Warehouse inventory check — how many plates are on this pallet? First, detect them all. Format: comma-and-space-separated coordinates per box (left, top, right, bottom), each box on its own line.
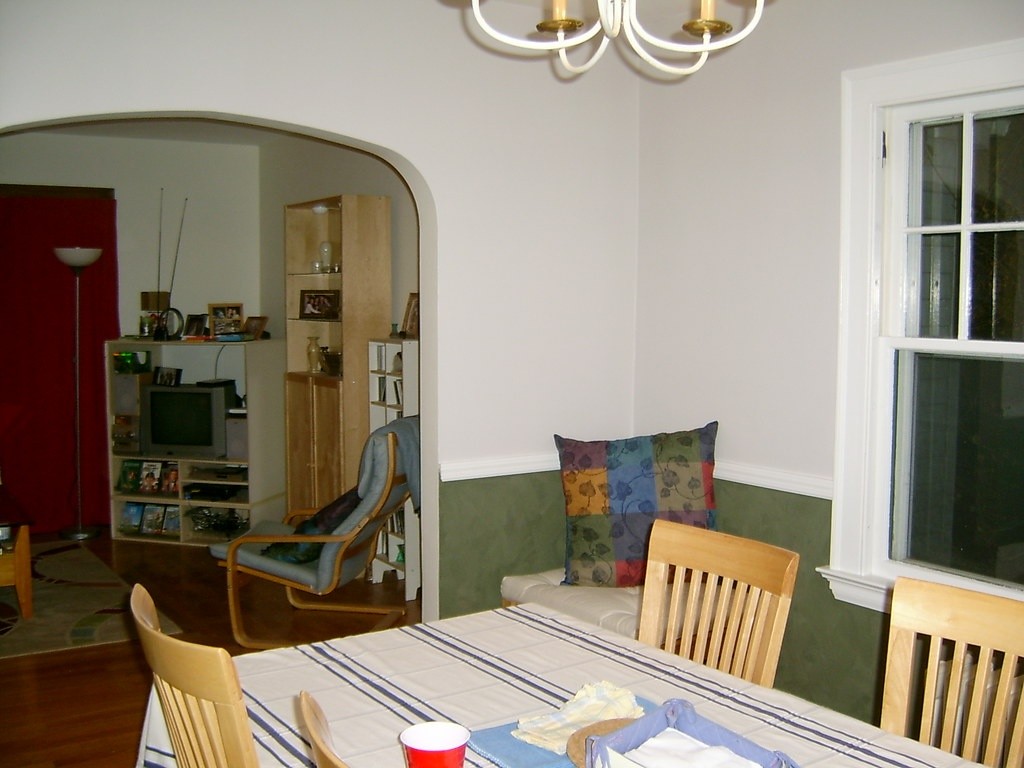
566, 718, 641, 767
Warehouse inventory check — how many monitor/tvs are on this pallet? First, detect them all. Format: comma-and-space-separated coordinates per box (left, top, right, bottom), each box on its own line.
141, 384, 227, 460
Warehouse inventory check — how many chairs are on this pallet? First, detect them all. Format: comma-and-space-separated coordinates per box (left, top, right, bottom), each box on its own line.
129, 584, 260, 768
210, 415, 420, 648
637, 520, 800, 688
880, 576, 1024, 768
299, 689, 350, 768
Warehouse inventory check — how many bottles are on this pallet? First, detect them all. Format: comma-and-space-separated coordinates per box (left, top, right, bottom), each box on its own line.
307, 337, 321, 372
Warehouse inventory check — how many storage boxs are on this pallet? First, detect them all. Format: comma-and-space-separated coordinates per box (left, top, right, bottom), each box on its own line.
113, 352, 152, 374
586, 698, 802, 768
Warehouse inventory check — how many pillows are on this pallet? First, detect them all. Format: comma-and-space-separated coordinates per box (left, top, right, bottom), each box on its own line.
554, 420, 718, 588
261, 484, 363, 565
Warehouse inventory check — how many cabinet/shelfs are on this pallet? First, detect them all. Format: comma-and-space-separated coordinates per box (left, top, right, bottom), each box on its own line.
283, 195, 393, 528
366, 338, 422, 600
104, 338, 283, 547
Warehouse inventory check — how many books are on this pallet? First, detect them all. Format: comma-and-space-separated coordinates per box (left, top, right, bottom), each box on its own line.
117, 461, 180, 537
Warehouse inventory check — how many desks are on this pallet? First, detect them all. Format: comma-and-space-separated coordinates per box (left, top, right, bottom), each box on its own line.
133, 601, 994, 767
0, 485, 35, 620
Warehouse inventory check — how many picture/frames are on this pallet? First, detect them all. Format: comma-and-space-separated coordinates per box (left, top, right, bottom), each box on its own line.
182, 314, 207, 337
243, 316, 269, 339
402, 293, 419, 340
208, 303, 244, 336
299, 289, 342, 320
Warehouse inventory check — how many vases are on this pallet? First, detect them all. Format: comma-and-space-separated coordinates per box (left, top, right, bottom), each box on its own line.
307, 337, 321, 373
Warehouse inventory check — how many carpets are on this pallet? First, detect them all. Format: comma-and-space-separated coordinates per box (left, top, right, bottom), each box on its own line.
0, 540, 182, 661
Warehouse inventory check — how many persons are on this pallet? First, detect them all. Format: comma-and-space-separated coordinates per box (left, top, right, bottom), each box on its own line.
159, 371, 174, 385
215, 309, 240, 332
304, 296, 333, 314
162, 468, 179, 493
140, 472, 157, 491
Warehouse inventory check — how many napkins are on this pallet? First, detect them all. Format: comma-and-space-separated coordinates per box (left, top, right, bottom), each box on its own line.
624, 728, 761, 767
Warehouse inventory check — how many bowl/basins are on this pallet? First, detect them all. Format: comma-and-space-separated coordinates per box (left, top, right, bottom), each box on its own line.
318, 352, 342, 375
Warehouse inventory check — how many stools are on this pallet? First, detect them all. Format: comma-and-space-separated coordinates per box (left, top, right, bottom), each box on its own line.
500, 567, 762, 660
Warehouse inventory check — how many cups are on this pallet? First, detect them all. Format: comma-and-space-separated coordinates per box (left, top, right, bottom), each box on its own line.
400, 722, 470, 768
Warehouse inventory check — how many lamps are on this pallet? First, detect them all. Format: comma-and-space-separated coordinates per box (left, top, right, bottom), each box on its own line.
312, 206, 328, 215
54, 247, 104, 543
140, 292, 170, 335
472, 0, 765, 76
